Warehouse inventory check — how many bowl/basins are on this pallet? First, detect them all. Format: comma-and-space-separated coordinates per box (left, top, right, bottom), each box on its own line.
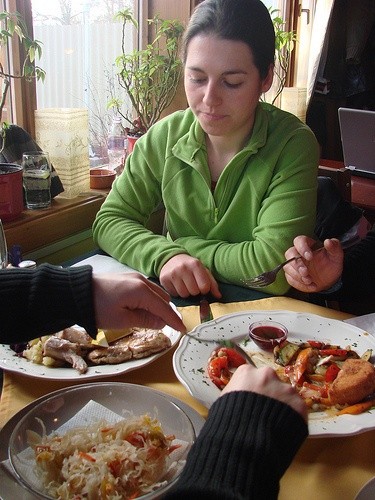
249, 321, 289, 350
90, 169, 116, 188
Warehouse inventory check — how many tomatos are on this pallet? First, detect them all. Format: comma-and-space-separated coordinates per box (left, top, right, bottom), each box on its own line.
208, 346, 246, 390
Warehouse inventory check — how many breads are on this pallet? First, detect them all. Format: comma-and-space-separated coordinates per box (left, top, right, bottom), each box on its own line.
18, 324, 171, 373
327, 358, 375, 404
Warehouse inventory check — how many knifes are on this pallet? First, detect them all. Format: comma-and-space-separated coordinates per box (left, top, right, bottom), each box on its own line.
199, 294, 214, 324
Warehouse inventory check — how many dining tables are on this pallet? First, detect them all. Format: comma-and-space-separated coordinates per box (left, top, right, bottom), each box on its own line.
0, 249, 375, 500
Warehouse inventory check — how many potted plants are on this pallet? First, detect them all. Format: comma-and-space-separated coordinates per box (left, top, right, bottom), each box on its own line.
106, 8, 186, 153
0, 10, 47, 221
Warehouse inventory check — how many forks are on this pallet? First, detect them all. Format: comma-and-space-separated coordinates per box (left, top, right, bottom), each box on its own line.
239, 226, 361, 288
181, 326, 258, 368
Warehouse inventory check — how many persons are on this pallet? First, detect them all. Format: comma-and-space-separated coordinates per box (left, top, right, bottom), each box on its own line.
283, 231, 375, 301
91, 0, 319, 297
0, 265, 309, 500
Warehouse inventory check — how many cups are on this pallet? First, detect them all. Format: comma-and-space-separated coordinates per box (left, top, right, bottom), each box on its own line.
22, 151, 51, 210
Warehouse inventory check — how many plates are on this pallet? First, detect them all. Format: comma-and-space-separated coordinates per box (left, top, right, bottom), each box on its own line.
1, 300, 185, 380
173, 309, 374, 440
0, 382, 209, 500
8, 381, 198, 500
